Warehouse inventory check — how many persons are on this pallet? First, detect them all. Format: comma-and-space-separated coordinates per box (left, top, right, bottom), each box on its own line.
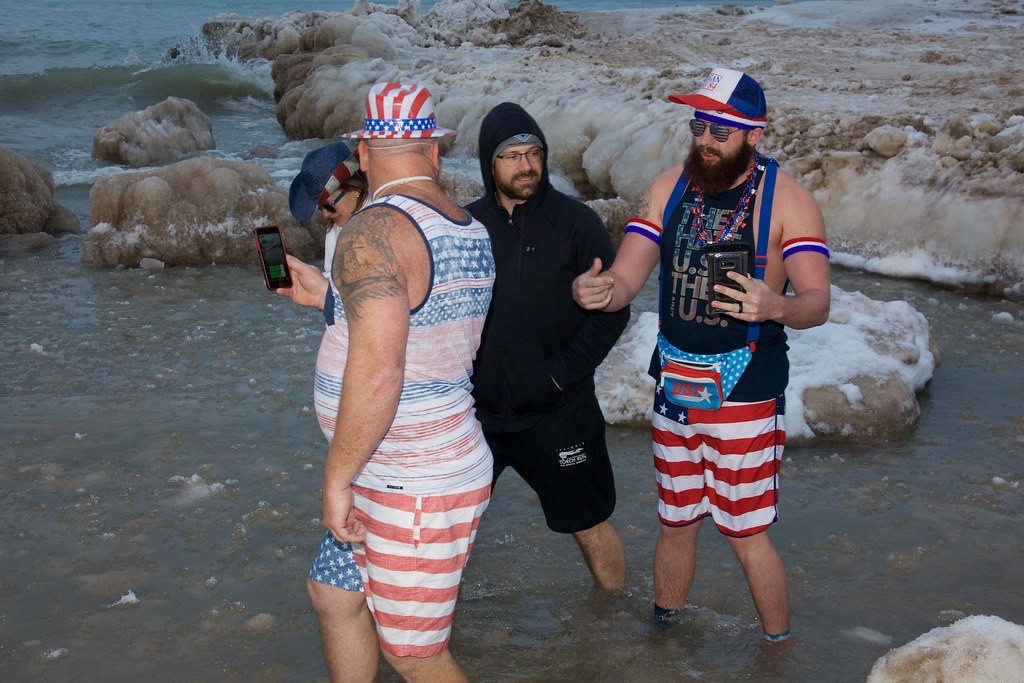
262, 77, 498, 683
456, 99, 634, 593
571, 67, 834, 645
289, 140, 378, 275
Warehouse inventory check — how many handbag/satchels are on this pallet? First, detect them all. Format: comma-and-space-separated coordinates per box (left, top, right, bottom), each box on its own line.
655, 332, 788, 412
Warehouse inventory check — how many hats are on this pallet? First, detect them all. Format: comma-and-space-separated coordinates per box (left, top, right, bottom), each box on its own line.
668, 68, 767, 119
340, 82, 458, 140
288, 144, 360, 222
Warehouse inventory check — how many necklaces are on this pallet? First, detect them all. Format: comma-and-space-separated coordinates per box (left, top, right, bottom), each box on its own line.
371, 173, 435, 203
688, 151, 761, 249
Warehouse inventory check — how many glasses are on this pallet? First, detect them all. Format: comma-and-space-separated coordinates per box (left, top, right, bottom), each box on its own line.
689, 119, 742, 143
497, 148, 544, 167
317, 190, 347, 214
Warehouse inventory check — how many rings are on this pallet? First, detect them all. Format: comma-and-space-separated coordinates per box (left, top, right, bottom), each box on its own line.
738, 302, 743, 314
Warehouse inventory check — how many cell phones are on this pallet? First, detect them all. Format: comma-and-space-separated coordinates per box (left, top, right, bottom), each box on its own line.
254, 226, 293, 290
708, 242, 753, 315
